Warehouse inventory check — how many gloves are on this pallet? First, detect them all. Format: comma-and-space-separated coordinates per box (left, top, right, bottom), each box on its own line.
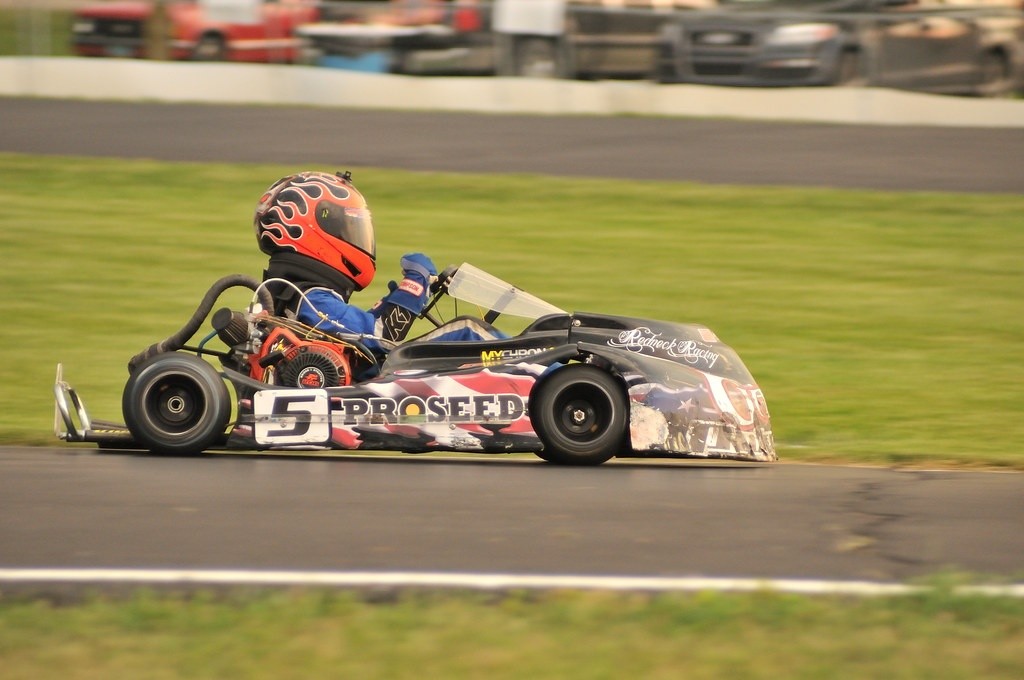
387, 253, 436, 316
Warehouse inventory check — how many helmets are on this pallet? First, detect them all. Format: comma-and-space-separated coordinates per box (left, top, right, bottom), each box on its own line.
253, 171, 376, 292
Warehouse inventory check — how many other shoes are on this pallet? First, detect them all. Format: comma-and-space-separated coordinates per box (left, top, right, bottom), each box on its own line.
585, 352, 612, 372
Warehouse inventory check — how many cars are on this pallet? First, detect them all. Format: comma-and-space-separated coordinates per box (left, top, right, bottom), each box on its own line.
70, 0, 446, 63
653, 0, 1022, 99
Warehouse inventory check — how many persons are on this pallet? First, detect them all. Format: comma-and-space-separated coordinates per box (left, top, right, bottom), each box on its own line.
254, 171, 513, 383
